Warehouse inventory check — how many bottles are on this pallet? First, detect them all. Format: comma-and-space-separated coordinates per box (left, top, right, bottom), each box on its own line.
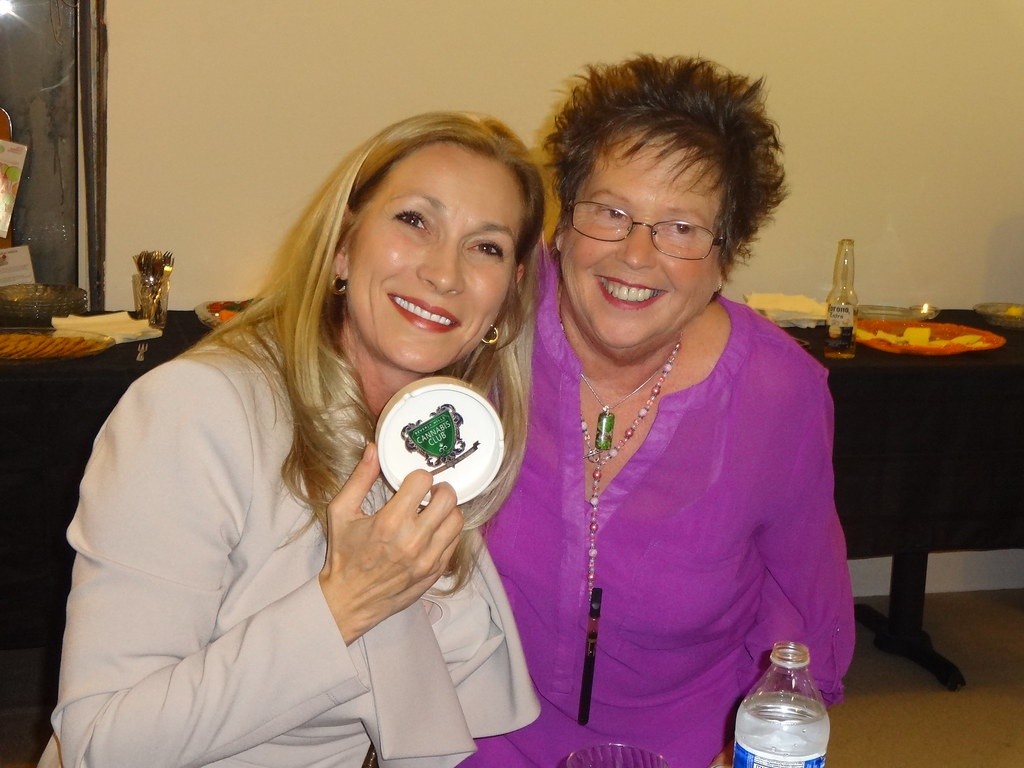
825, 237, 861, 359
731, 639, 830, 768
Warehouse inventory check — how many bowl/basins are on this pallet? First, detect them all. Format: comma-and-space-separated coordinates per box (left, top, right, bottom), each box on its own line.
909, 305, 941, 320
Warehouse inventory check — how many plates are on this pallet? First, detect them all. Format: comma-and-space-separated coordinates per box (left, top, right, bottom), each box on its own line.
193, 299, 230, 329
0, 283, 89, 327
856, 304, 925, 322
973, 302, 1023, 327
1, 327, 117, 366
856, 317, 1008, 355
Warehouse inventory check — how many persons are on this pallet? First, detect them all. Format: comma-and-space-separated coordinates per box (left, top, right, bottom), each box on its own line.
417, 50, 857, 768
37, 105, 547, 768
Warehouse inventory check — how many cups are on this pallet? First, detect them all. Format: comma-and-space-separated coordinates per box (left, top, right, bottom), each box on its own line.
564, 744, 670, 767
132, 272, 169, 329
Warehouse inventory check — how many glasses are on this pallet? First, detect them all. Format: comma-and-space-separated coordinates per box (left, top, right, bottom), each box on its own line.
566, 200, 726, 260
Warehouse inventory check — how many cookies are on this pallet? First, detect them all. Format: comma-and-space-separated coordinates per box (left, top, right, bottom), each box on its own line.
0, 334, 108, 358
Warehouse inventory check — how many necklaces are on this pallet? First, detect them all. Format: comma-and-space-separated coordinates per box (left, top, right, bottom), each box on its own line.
544, 281, 686, 625
563, 357, 665, 460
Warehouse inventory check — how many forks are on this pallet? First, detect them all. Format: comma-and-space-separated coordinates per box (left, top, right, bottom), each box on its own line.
132, 249, 175, 327
136, 343, 149, 361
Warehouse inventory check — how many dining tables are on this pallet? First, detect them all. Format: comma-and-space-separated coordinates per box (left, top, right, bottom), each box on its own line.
0, 304, 1023, 693
